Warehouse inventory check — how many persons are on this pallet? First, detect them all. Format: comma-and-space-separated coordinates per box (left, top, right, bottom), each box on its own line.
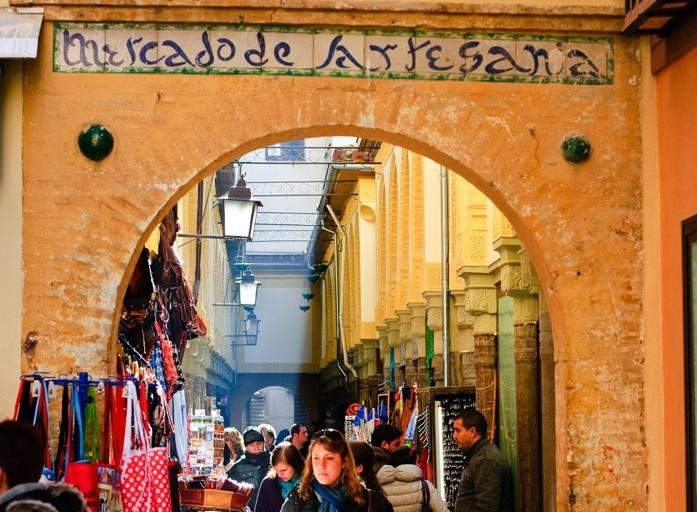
223, 423, 451, 512
0, 420, 90, 512
453, 409, 513, 512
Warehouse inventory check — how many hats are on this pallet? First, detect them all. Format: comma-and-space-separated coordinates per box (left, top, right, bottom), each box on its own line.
243, 425, 265, 445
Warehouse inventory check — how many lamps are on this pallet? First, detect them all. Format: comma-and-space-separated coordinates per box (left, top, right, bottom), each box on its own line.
176, 173, 264, 250
230, 335, 257, 348
224, 310, 261, 338
213, 267, 261, 312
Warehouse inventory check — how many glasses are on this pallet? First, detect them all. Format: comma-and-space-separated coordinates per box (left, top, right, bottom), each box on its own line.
313, 428, 342, 443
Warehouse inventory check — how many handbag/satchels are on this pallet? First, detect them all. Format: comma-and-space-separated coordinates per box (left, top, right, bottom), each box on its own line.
16, 317, 185, 512
421, 479, 435, 511
